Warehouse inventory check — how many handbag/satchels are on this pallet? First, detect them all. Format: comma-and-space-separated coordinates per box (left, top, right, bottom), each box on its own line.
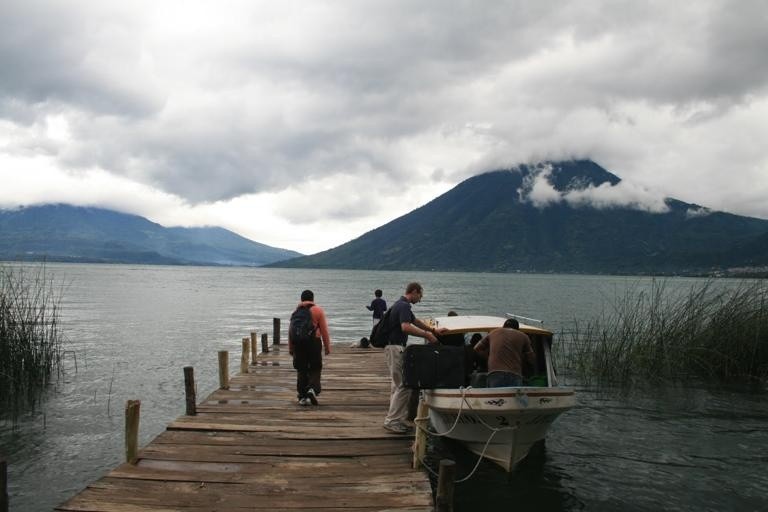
401, 345, 467, 391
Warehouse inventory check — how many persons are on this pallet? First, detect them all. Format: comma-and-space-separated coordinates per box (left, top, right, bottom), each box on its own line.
440, 308, 464, 347
472, 318, 538, 389
381, 281, 450, 435
287, 288, 332, 408
456, 333, 487, 374
363, 288, 388, 328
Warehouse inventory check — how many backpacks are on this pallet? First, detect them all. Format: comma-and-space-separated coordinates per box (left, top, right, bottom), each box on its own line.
289, 303, 320, 342
369, 299, 401, 348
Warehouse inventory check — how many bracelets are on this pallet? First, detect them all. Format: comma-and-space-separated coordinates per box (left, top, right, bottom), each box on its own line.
431, 327, 435, 333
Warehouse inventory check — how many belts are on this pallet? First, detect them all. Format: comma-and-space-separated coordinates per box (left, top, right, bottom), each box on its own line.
386, 341, 406, 347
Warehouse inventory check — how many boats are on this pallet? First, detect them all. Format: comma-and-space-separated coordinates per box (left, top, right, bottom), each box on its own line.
423, 314, 579, 470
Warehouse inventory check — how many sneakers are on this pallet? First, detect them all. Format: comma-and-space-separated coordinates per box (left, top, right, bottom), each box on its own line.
296, 397, 307, 406
307, 388, 319, 406
383, 416, 413, 434
